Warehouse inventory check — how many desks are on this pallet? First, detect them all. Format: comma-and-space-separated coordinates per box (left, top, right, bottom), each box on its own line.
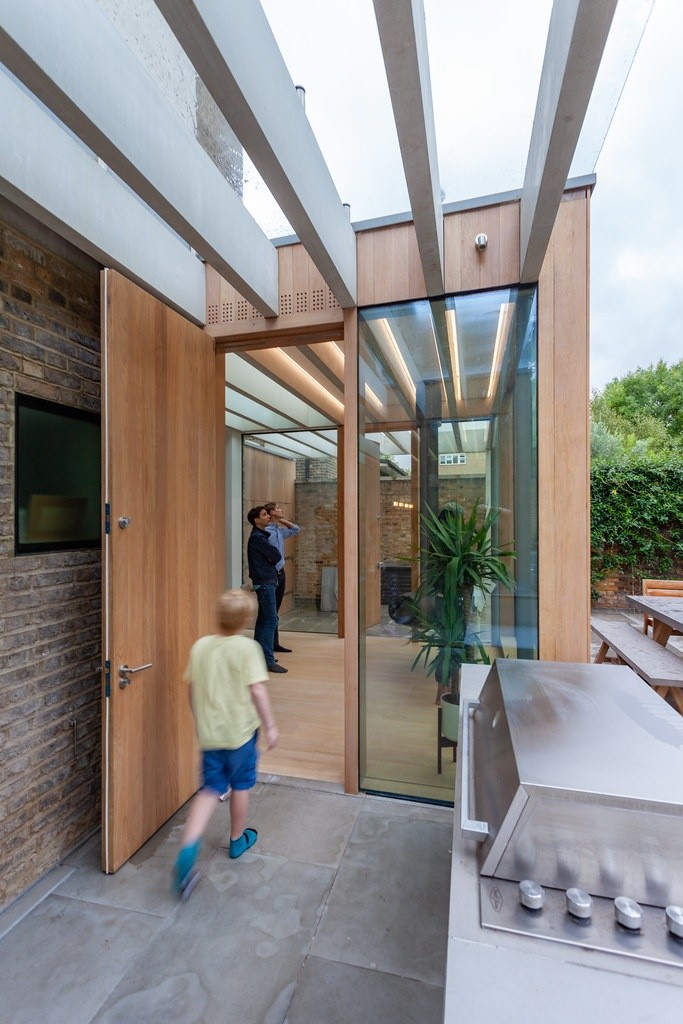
626, 595, 683, 647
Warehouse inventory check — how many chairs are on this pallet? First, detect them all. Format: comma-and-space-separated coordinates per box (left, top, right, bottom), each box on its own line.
643, 579, 683, 635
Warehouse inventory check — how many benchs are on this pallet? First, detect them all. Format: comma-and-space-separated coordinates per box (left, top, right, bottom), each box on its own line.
590, 620, 683, 714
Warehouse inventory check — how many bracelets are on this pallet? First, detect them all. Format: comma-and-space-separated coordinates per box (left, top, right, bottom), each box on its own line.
264, 722, 277, 732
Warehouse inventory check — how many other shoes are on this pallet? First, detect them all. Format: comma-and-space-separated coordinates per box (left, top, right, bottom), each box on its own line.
275, 646, 292, 652
274, 659, 278, 662
268, 664, 288, 673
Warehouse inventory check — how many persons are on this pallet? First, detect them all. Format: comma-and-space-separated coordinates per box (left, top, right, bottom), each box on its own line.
247, 506, 288, 673
169, 587, 279, 895
264, 503, 300, 662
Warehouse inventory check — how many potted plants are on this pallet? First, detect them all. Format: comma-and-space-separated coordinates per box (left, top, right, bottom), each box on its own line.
421, 506, 490, 774
